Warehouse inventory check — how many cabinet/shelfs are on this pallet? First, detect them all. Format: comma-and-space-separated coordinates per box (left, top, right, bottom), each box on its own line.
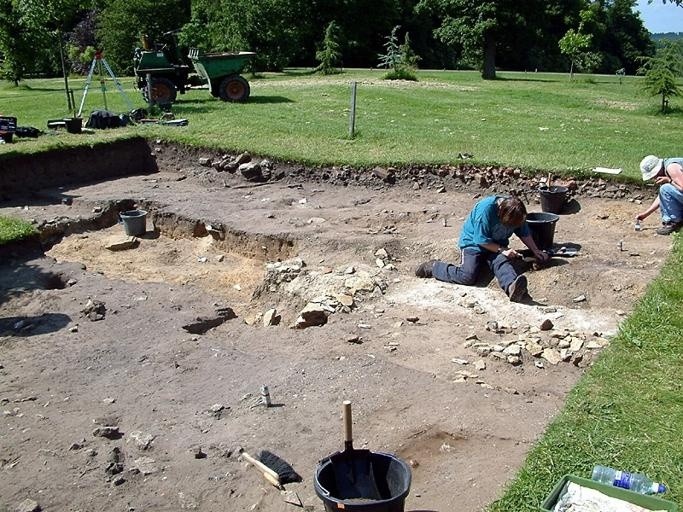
590, 464, 667, 497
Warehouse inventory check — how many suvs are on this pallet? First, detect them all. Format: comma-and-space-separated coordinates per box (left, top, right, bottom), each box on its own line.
118, 209, 149, 238
312, 449, 413, 511
525, 211, 560, 252
538, 184, 569, 214
64, 109, 84, 132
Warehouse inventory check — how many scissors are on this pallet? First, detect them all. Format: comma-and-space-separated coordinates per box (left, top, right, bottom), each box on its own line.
263, 472, 301, 506
328, 400, 382, 500
516, 253, 539, 263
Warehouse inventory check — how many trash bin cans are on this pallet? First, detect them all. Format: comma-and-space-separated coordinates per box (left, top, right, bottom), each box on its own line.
496, 242, 502, 255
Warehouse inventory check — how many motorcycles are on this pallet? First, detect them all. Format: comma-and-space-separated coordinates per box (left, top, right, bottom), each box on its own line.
77, 51, 135, 117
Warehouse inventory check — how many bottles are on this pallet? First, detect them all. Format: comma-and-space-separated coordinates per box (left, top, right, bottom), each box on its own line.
640, 154, 663, 181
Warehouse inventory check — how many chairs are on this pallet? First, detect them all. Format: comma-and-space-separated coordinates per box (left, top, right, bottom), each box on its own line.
416, 258, 441, 278
656, 221, 681, 235
508, 274, 528, 303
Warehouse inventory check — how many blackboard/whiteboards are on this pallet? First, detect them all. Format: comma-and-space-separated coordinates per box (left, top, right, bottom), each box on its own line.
130, 27, 261, 113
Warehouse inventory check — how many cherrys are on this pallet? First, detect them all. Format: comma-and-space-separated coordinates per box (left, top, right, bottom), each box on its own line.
635, 209, 640, 231
241, 450, 302, 484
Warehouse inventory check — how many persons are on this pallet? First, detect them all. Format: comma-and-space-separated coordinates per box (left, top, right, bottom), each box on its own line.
413, 194, 551, 303
633, 154, 683, 236
